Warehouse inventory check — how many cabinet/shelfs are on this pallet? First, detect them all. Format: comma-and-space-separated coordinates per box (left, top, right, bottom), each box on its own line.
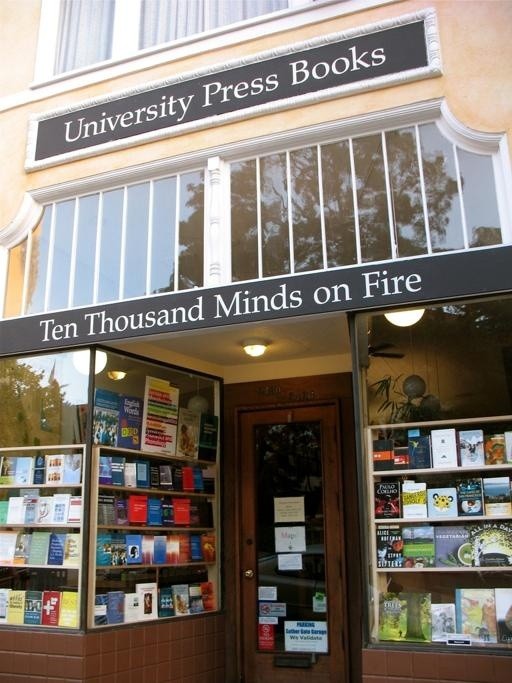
84, 444, 222, 631
363, 415, 512, 649
0, 443, 84, 630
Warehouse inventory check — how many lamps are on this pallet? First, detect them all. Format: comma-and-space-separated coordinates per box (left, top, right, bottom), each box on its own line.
385, 308, 424, 327
242, 337, 266, 357
74, 349, 107, 376
109, 371, 126, 380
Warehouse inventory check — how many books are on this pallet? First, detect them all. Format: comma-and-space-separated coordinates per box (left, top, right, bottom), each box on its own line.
0, 493, 82, 525
95, 581, 217, 626
1, 530, 80, 564
374, 476, 512, 517
0, 586, 78, 629
0, 453, 83, 488
373, 427, 512, 470
378, 587, 511, 646
92, 374, 217, 566
376, 525, 512, 569
